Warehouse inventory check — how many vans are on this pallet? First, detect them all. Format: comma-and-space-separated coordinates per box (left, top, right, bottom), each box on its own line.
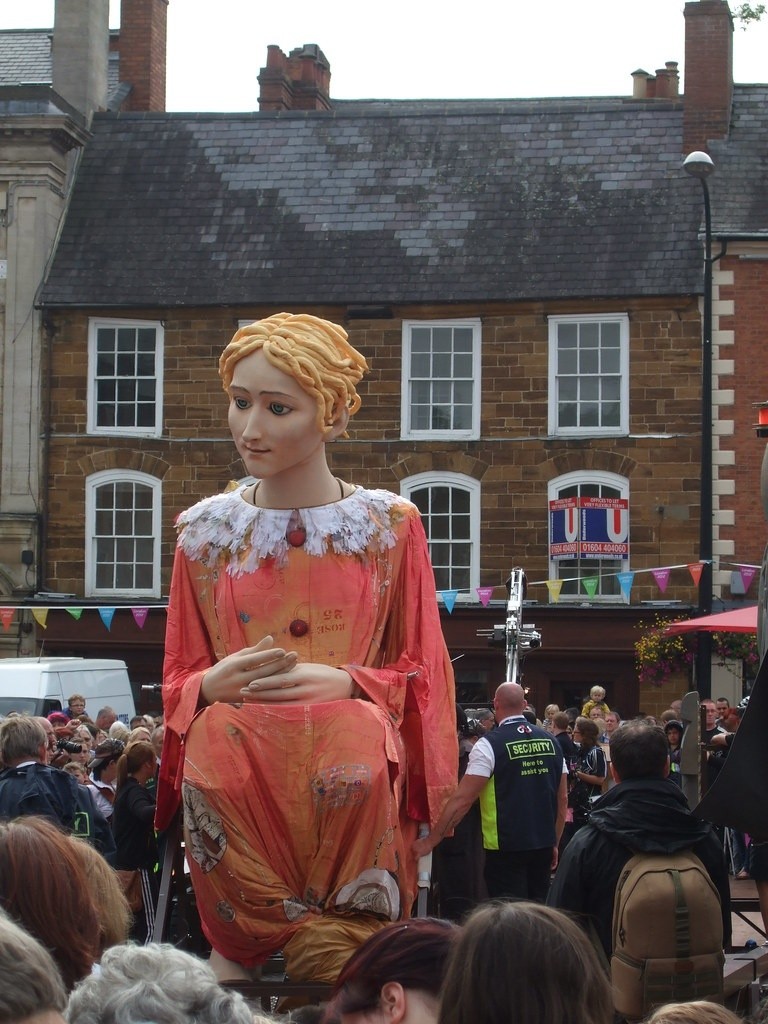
0, 657, 135, 730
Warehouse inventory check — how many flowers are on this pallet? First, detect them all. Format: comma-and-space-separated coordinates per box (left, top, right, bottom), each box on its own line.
712, 628, 761, 681
635, 608, 703, 685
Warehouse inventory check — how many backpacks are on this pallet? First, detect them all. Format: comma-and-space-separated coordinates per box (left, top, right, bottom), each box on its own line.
611, 850, 726, 1016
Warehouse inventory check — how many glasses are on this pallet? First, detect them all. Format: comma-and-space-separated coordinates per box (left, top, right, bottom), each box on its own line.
573, 731, 580, 734
69, 704, 84, 707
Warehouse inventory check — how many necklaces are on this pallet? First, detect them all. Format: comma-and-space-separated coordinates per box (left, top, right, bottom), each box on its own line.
252, 478, 344, 507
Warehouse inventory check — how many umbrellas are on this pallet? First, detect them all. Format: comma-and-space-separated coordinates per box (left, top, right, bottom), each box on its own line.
663, 604, 757, 637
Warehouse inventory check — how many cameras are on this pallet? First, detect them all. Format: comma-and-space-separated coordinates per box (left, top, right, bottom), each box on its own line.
56, 738, 82, 754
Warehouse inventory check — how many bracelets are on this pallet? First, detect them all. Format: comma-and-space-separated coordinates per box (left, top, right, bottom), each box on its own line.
555, 843, 559, 848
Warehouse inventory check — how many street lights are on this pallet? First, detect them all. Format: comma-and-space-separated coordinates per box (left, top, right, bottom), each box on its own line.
680, 150, 728, 706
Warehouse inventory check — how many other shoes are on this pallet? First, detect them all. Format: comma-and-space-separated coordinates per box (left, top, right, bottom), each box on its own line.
735, 872, 750, 879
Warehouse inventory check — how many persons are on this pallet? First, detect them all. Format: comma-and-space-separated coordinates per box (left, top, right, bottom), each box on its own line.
547, 718, 731, 1024
152, 310, 456, 1012
0, 684, 768, 1024
437, 898, 615, 1024
413, 681, 569, 905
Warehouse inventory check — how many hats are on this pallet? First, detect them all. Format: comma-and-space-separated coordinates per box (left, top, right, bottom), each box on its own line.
736, 695, 750, 712
47, 711, 70, 724
86, 739, 126, 768
